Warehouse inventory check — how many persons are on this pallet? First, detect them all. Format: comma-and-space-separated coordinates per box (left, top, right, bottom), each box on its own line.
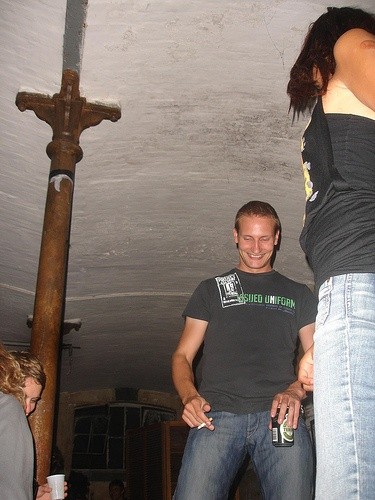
286, 6, 375, 500
8, 351, 68, 500
171, 200, 317, 500
109, 479, 125, 500
0, 344, 35, 500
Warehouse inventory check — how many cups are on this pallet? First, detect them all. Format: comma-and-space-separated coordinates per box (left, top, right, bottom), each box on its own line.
47, 475, 64, 500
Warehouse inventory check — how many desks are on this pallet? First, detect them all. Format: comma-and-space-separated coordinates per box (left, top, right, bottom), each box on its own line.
125, 420, 241, 500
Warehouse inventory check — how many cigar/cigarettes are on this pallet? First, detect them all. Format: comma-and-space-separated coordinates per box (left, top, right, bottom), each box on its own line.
197, 417, 212, 431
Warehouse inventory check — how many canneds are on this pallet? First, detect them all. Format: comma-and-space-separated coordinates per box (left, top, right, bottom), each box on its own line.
272, 407, 294, 447
